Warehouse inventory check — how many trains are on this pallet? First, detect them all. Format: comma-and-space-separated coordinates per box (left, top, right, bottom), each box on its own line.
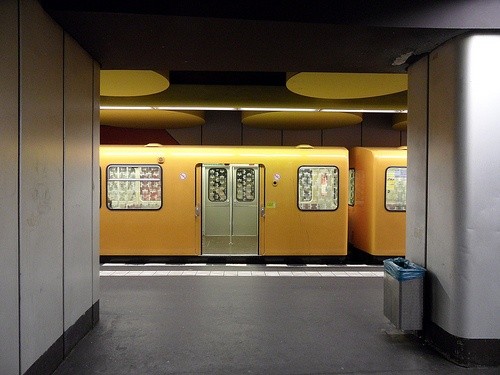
98, 140, 409, 266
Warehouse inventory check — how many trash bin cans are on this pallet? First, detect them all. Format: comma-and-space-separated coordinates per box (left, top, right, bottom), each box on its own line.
383, 257, 428, 332
